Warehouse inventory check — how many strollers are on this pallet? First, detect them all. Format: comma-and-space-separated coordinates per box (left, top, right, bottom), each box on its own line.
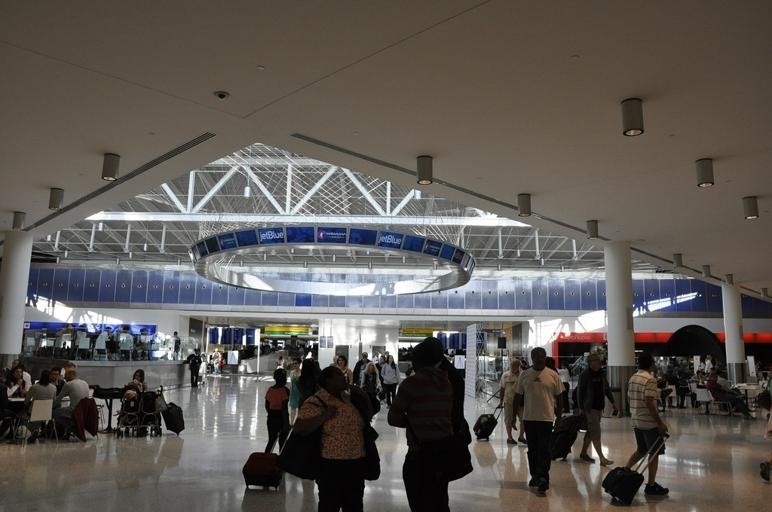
114, 383, 167, 439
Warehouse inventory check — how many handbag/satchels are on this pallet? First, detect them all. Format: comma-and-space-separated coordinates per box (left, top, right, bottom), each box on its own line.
407, 431, 474, 486
276, 426, 321, 481
363, 427, 380, 481
554, 414, 589, 432
757, 389, 772, 412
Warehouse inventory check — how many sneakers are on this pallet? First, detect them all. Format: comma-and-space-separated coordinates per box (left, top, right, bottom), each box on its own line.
518, 437, 527, 444
758, 462, 770, 481
660, 401, 702, 409
528, 478, 548, 498
644, 482, 670, 497
27, 429, 46, 444
580, 452, 596, 463
599, 459, 615, 467
507, 438, 517, 445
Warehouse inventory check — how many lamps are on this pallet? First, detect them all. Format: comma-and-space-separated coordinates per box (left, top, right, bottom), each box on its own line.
433, 258, 438, 270
726, 274, 733, 286
49, 188, 64, 210
244, 145, 251, 199
101, 153, 120, 181
239, 247, 406, 269
702, 264, 710, 277
620, 98, 644, 136
673, 254, 682, 266
742, 196, 759, 220
761, 288, 767, 297
12, 212, 24, 229
586, 220, 598, 239
46, 222, 181, 266
498, 248, 562, 272
416, 155, 432, 186
517, 193, 531, 217
695, 158, 714, 188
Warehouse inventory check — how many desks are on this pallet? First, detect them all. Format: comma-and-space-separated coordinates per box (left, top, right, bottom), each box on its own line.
92, 387, 125, 434
734, 382, 760, 412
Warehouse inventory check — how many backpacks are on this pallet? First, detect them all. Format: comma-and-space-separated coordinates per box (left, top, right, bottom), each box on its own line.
571, 370, 608, 411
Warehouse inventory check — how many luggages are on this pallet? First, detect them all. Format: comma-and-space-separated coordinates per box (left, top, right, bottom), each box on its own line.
160, 402, 186, 436
241, 451, 283, 491
551, 431, 578, 462
473, 413, 497, 440
377, 389, 386, 403
602, 466, 644, 505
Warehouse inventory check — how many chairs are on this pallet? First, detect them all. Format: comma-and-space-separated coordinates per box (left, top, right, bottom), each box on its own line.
0, 396, 95, 448
657, 382, 735, 417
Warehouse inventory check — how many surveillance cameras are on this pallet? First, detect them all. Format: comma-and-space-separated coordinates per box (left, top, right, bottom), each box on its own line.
214, 91, 229, 101
